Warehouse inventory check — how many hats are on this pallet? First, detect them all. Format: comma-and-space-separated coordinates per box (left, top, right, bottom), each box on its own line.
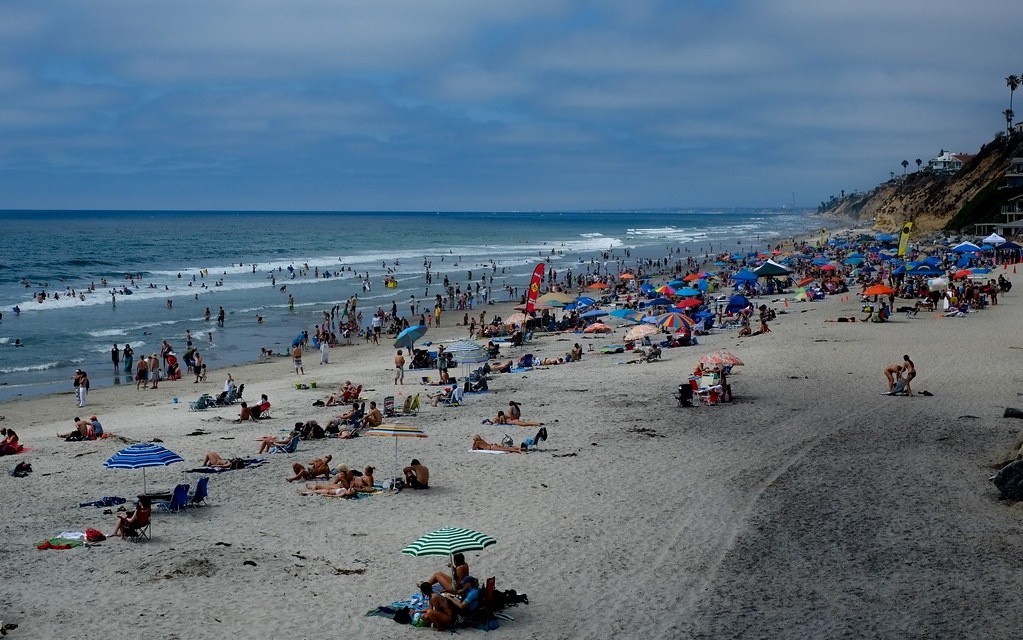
140, 355, 144, 358
89, 415, 97, 420
436, 389, 440, 393
342, 383, 349, 388
452, 384, 458, 388
444, 387, 450, 391
167, 351, 177, 356
75, 369, 81, 373
148, 355, 151, 358
151, 352, 159, 359
338, 463, 348, 472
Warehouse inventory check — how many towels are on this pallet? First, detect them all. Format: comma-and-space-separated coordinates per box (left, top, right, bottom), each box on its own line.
690, 318, 705, 332
32, 531, 109, 551
880, 379, 907, 396
451, 387, 464, 403
491, 337, 512, 342
511, 367, 534, 373
467, 448, 510, 455
361, 484, 389, 495
363, 582, 445, 628
339, 492, 374, 501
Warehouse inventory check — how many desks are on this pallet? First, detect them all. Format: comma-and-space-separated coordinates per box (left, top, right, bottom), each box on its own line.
138, 492, 171, 503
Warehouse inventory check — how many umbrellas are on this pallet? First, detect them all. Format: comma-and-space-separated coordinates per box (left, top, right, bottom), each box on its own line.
101, 233, 1023, 585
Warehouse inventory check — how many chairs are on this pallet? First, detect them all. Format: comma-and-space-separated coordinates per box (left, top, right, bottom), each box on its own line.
442, 387, 463, 407
187, 476, 210, 508
426, 351, 439, 366
520, 426, 547, 451
332, 385, 363, 404
269, 430, 302, 453
380, 394, 420, 417
455, 577, 495, 629
123, 499, 153, 542
157, 484, 191, 515
509, 295, 602, 344
487, 343, 500, 358
518, 352, 533, 371
675, 363, 735, 406
188, 383, 270, 419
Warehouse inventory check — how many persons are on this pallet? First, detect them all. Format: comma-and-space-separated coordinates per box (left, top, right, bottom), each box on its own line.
107, 496, 149, 537
413, 553, 480, 628
0, 233, 1023, 499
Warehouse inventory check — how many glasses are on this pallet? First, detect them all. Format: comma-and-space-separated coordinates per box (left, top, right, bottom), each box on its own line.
325, 455, 331, 461
